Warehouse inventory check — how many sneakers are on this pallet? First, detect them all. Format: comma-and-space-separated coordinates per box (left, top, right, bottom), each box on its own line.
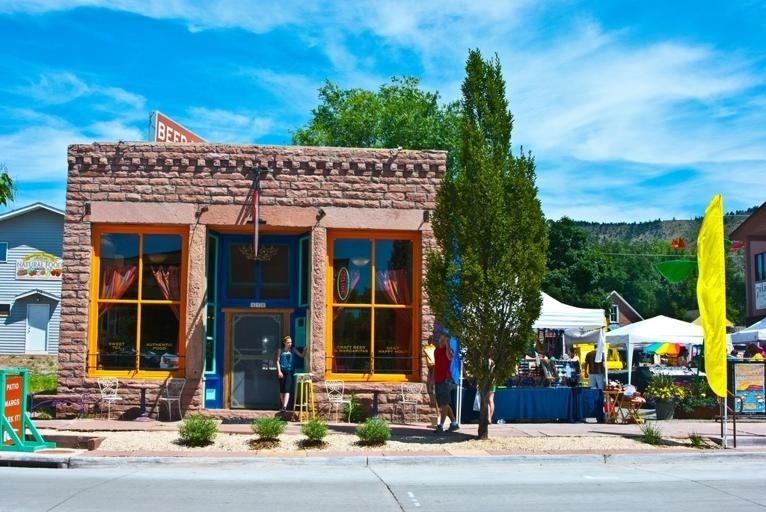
279, 407, 287, 411
446, 423, 459, 433
435, 425, 443, 431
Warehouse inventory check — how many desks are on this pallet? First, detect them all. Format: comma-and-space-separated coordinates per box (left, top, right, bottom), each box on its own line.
124, 382, 156, 421
358, 386, 394, 421
603, 389, 644, 425
462, 386, 603, 423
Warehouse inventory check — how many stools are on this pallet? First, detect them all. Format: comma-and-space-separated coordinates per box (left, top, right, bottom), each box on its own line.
293, 372, 318, 422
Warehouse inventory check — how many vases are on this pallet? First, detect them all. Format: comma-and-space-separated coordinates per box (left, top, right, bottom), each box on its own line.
653, 396, 675, 420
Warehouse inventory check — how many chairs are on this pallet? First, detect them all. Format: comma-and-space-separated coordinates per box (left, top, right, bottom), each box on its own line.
326, 380, 351, 424
98, 376, 123, 419
393, 382, 422, 424
157, 376, 190, 421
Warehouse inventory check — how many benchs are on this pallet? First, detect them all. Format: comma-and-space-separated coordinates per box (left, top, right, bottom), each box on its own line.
29, 393, 90, 418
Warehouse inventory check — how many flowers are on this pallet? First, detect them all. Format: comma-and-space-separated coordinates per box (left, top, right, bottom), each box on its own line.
642, 369, 685, 403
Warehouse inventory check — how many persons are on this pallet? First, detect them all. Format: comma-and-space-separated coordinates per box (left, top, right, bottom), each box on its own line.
433, 331, 460, 434
274, 334, 308, 413
567, 348, 578, 362
475, 355, 497, 424
584, 343, 607, 390
728, 342, 765, 362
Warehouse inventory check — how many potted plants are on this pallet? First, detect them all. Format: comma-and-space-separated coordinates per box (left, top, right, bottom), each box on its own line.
674, 340, 720, 419
